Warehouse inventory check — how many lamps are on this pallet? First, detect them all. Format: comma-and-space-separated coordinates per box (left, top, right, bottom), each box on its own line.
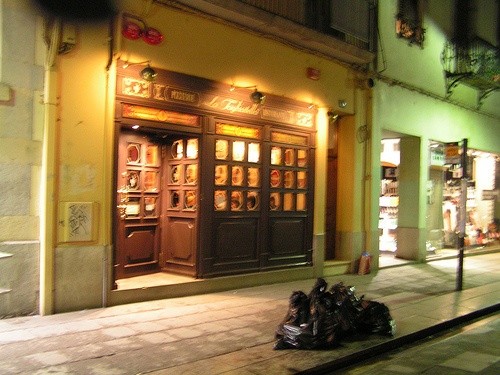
122, 59, 158, 82
230, 84, 264, 105
307, 103, 339, 125
